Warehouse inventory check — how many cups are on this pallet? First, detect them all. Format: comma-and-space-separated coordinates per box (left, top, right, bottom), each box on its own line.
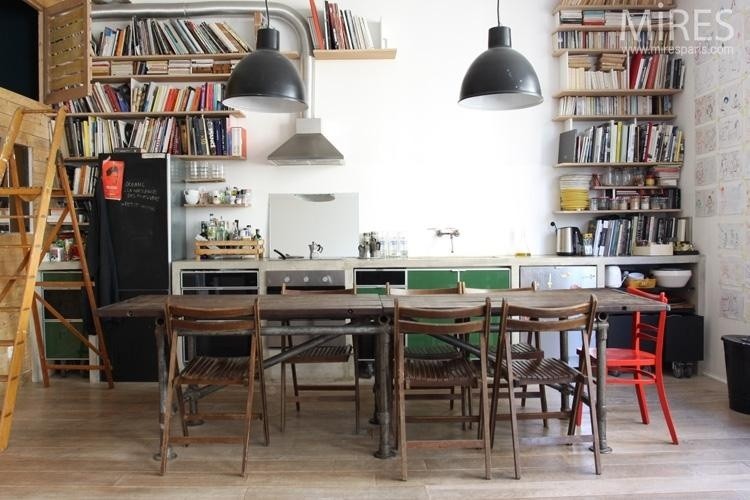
628, 272, 645, 279
596, 166, 646, 186
185, 160, 224, 179
183, 189, 211, 205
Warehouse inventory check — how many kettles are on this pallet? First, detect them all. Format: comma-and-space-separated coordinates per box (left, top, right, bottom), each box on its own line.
556, 226, 582, 257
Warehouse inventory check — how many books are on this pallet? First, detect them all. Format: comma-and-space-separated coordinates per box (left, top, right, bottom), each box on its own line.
553, 0, 693, 256
46, 158, 126, 225
54, 77, 246, 157
46, 1, 384, 76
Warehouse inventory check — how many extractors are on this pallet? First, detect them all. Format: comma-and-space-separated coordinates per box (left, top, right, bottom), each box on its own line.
267, 116, 346, 166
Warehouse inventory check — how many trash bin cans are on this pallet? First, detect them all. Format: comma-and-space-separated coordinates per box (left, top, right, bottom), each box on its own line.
720, 335, 750, 415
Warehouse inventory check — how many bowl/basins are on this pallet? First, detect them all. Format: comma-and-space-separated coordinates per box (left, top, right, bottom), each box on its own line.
649, 268, 693, 288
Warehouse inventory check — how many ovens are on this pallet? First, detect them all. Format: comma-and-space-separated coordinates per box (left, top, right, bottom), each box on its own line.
268, 286, 348, 348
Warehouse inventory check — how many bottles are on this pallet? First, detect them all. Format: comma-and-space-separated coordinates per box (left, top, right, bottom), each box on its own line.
646, 171, 656, 185
605, 266, 622, 289
199, 213, 262, 260
360, 232, 380, 257
213, 185, 249, 205
582, 233, 594, 255
591, 195, 667, 211
381, 235, 408, 258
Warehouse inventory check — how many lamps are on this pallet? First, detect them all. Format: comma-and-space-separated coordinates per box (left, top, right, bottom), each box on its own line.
455, 1, 544, 112
220, 0, 309, 114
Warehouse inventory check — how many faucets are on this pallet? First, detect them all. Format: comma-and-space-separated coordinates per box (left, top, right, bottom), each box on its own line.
435, 228, 459, 254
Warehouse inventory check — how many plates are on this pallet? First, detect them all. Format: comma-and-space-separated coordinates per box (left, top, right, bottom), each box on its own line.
559, 171, 591, 210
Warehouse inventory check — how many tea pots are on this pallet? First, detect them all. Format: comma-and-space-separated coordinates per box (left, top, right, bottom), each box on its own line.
308, 242, 320, 260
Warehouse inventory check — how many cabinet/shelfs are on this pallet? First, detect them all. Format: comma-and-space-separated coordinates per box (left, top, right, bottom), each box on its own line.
50, 4, 303, 260
551, 9, 694, 218
351, 262, 703, 369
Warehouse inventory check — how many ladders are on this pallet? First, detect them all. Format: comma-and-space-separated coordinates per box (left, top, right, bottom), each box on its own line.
0, 106, 65, 453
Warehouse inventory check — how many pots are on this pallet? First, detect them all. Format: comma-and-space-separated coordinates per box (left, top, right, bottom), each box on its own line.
273, 249, 304, 259
